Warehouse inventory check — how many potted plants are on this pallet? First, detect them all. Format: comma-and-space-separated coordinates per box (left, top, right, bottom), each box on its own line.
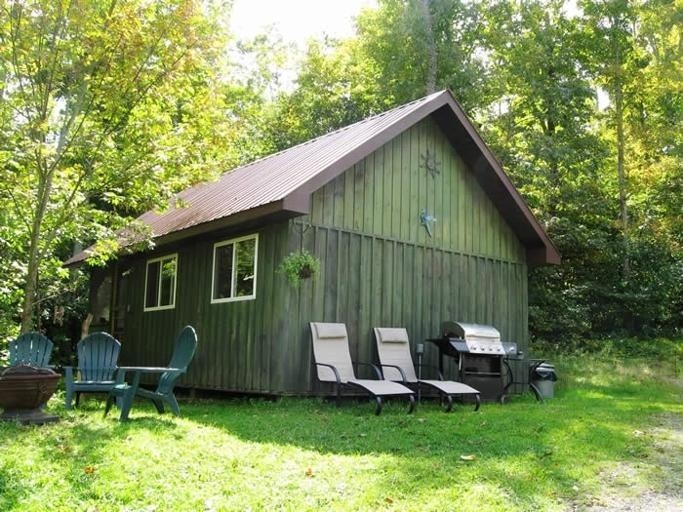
274, 247, 320, 289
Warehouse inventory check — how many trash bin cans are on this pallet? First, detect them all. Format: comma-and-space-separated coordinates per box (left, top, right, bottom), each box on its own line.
531, 363, 556, 399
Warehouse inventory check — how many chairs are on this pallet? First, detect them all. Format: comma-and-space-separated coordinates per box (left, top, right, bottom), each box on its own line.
62, 324, 198, 425
8, 333, 55, 408
308, 321, 480, 415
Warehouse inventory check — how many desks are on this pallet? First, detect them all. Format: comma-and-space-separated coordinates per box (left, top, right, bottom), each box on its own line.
500, 358, 550, 405
